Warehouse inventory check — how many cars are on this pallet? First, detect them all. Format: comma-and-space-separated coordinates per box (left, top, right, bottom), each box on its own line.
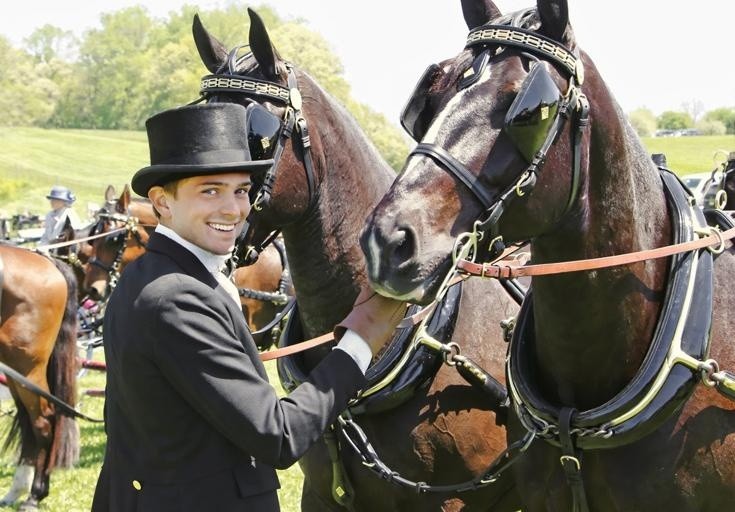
678, 169, 723, 206
652, 128, 701, 137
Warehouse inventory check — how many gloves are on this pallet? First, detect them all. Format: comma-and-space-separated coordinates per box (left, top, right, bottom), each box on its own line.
330, 269, 410, 364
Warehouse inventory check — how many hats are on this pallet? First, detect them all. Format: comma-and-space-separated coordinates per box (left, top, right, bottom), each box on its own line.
131, 102, 278, 197
44, 185, 78, 205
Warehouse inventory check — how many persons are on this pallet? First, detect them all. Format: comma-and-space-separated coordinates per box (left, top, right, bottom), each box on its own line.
91, 100, 405, 511
38, 185, 81, 246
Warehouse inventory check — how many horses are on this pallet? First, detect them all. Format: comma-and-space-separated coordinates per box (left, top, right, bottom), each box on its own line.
52, 215, 100, 301
0, 242, 85, 512
82, 184, 294, 352
185, 7, 531, 511
358, 0, 734, 512
